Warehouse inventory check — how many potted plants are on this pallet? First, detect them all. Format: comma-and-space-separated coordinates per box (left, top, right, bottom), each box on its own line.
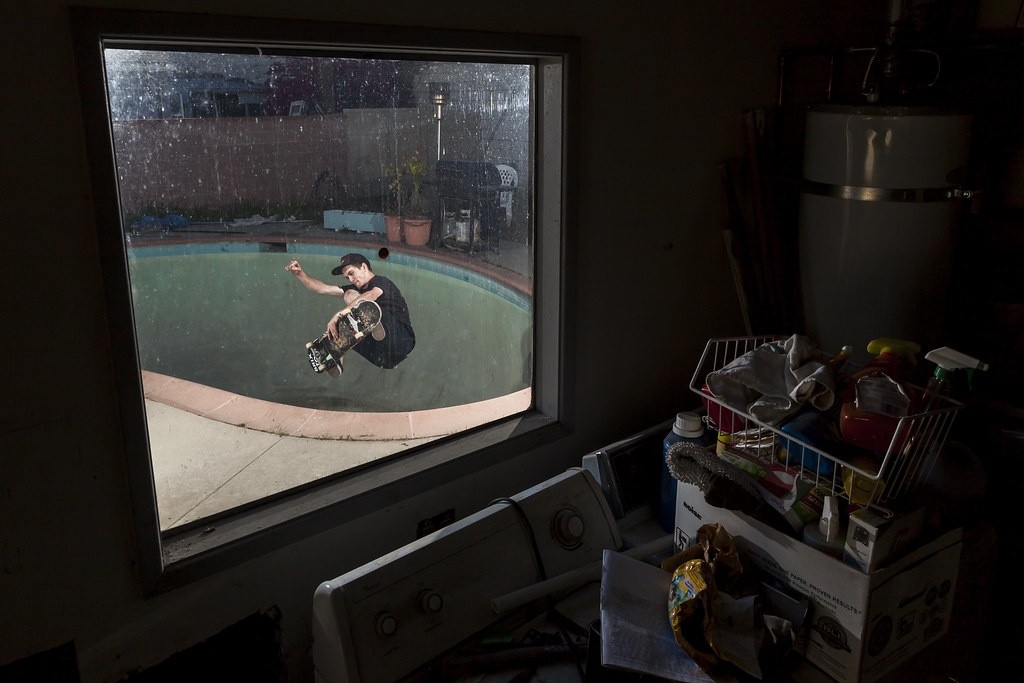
402, 154, 433, 248
382, 164, 403, 242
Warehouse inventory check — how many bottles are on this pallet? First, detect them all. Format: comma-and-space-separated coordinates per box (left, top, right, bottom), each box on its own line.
660, 412, 704, 529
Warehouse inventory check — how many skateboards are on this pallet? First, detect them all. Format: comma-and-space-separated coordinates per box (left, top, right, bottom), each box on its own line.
304, 299, 383, 374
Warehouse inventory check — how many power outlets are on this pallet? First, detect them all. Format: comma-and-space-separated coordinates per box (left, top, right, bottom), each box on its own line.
417, 508, 456, 540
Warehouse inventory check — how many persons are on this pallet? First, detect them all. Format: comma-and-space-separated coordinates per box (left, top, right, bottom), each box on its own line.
285, 252, 415, 378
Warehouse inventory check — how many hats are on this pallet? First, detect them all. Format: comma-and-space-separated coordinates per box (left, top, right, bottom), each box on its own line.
331, 253, 370, 275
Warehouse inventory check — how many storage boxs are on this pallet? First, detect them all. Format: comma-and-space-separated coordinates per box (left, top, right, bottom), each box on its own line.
673, 478, 964, 683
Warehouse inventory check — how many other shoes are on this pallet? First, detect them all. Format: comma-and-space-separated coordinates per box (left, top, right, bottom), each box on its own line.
355, 300, 386, 341
325, 360, 344, 378
